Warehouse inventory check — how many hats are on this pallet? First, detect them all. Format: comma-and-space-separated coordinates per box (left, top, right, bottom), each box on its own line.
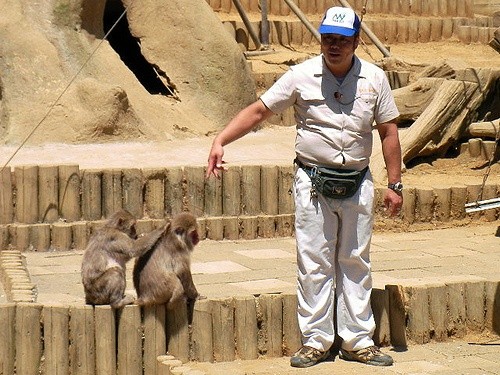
319, 6, 361, 36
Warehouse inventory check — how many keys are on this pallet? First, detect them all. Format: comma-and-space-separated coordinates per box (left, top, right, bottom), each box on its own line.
309, 165, 320, 202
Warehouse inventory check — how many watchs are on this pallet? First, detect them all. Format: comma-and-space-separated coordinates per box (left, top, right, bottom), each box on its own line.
388, 182, 403, 193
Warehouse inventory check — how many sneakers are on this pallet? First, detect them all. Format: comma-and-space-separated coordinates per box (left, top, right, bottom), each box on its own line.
339, 345, 393, 366
290, 346, 331, 368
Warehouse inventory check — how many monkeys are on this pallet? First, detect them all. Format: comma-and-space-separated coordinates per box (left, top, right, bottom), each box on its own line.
81, 209, 208, 310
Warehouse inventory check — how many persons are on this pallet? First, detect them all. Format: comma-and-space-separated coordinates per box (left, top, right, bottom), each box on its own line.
206, 5, 403, 367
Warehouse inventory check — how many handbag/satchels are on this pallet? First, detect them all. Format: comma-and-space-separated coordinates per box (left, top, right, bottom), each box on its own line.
293, 157, 369, 199
